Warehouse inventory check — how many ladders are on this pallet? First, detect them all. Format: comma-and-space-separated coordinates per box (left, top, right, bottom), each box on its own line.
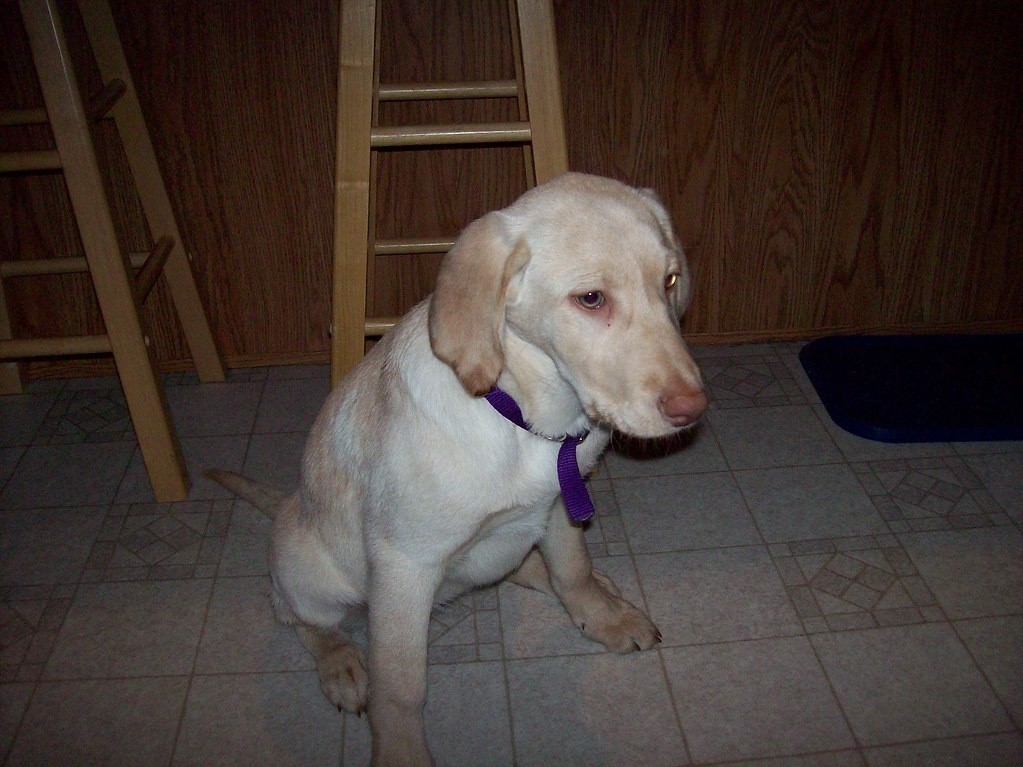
0, 1, 227, 502
333, 0, 568, 390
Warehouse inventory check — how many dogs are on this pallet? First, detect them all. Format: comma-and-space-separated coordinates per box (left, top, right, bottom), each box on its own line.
198, 171, 710, 767
